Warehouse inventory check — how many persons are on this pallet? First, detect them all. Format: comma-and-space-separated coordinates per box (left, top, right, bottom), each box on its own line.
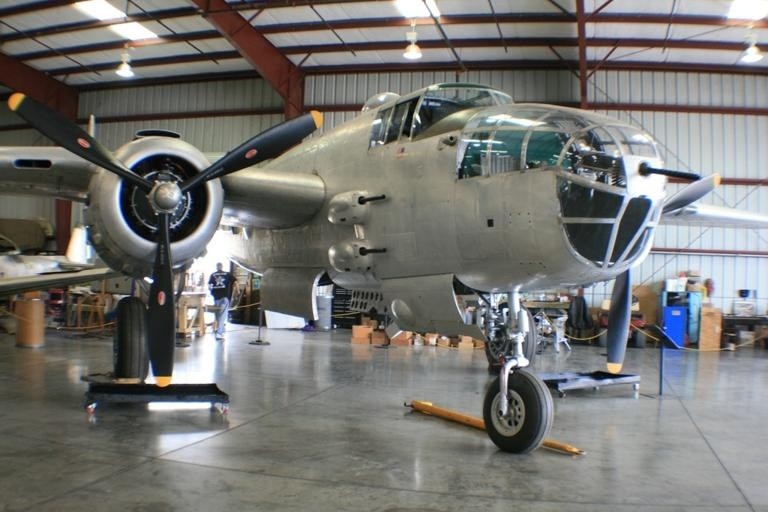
566, 287, 594, 346
207, 263, 241, 341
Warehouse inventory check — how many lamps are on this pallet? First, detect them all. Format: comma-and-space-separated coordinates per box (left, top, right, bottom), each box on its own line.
403, 22, 422, 59
740, 24, 763, 62
116, 42, 134, 78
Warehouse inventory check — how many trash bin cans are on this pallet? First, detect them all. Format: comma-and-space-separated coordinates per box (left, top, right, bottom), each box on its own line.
312, 295, 334, 330
13, 298, 46, 348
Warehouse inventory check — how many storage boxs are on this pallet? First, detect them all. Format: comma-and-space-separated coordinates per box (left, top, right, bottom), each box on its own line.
352, 317, 485, 349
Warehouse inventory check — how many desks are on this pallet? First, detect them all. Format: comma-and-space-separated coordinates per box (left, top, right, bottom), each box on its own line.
174, 291, 206, 347
721, 314, 768, 351
